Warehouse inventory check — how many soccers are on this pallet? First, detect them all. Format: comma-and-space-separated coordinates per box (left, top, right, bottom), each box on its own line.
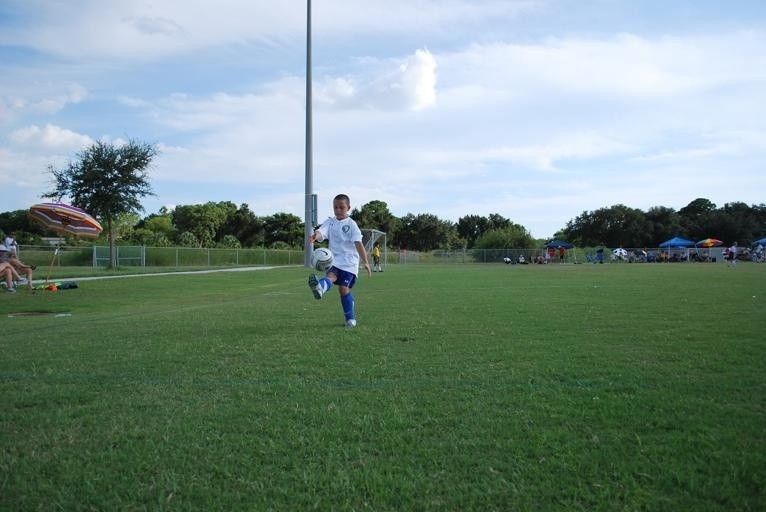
311, 247, 333, 272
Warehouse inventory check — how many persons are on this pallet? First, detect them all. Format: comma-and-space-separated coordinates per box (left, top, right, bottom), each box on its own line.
0, 231, 36, 292
371, 241, 384, 272
307, 193, 372, 329
595, 241, 766, 267
503, 246, 566, 265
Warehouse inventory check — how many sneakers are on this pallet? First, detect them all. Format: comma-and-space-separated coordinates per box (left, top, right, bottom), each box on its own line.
308, 274, 323, 299
345, 318, 356, 328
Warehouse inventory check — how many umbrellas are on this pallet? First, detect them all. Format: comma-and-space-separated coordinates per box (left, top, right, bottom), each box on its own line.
659, 236, 696, 247
695, 238, 724, 247
546, 239, 574, 249
752, 238, 766, 245
30, 201, 104, 294
